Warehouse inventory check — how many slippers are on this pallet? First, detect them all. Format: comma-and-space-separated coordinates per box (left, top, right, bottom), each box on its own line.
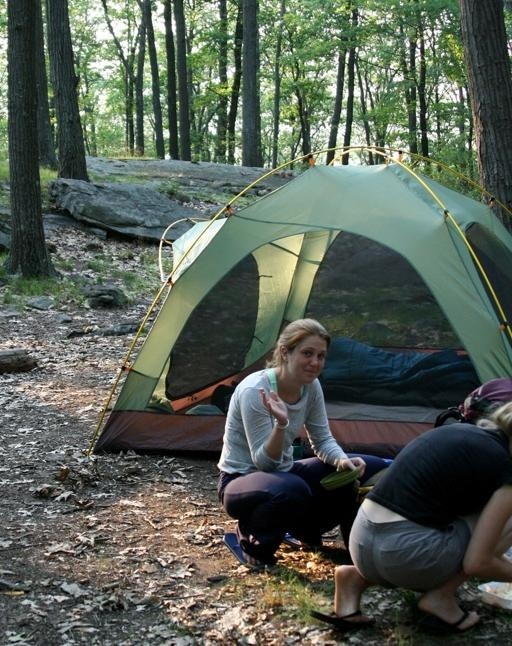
223, 533, 271, 568
403, 608, 479, 636
282, 532, 302, 548
310, 609, 376, 630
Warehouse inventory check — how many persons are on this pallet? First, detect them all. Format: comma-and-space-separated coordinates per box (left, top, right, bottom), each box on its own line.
304, 399, 512, 640
217, 317, 392, 572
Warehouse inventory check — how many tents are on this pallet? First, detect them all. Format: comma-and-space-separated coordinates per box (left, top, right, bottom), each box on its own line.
83, 138, 511, 467
